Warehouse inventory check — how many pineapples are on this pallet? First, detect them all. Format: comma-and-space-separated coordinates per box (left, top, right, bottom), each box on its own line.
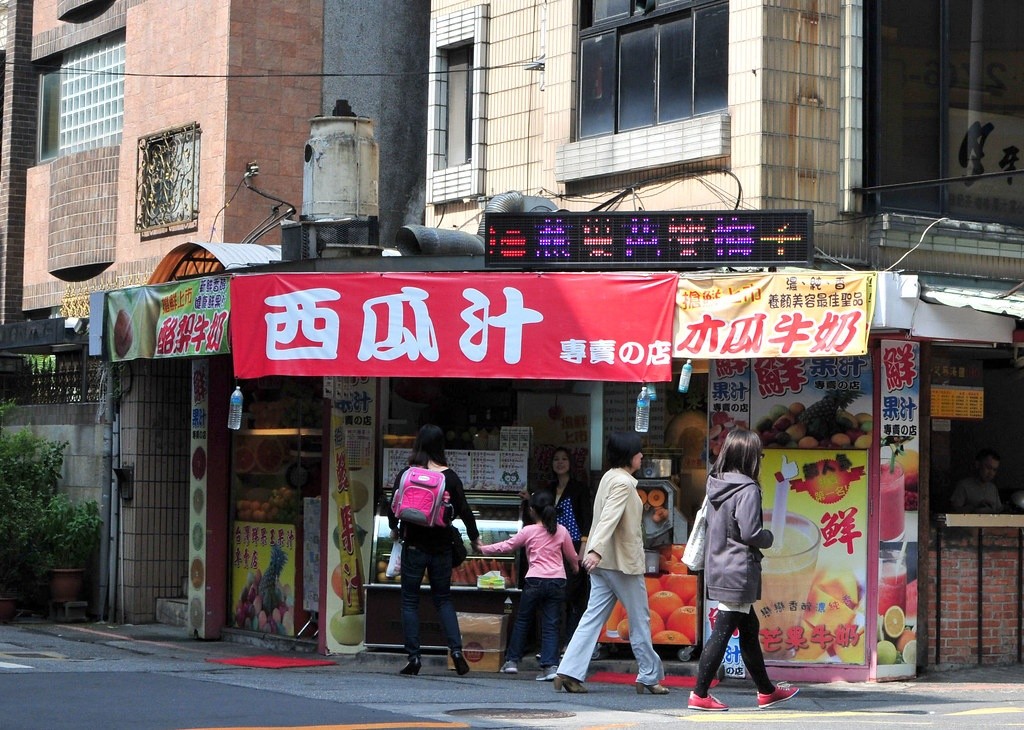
798, 389, 865, 427
260, 542, 288, 592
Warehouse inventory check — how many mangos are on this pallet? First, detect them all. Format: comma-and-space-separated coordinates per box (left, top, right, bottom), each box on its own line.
792, 567, 867, 662
754, 402, 871, 449
235, 571, 294, 635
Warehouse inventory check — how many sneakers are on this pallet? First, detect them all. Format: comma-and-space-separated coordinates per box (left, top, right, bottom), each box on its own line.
757, 686, 799, 708
500, 661, 518, 673
536, 666, 558, 680
688, 691, 729, 711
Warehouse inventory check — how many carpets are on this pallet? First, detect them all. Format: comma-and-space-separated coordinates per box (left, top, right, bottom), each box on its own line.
206, 655, 335, 669
584, 670, 720, 688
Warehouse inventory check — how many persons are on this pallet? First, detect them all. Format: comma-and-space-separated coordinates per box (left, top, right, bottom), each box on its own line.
519, 446, 592, 658
470, 491, 580, 681
949, 450, 1008, 514
687, 428, 799, 710
554, 432, 669, 695
387, 423, 482, 676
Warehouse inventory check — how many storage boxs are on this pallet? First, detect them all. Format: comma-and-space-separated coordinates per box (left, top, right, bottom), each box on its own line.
448, 611, 509, 672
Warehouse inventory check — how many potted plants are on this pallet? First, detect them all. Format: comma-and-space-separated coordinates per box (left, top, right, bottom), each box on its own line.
0, 399, 104, 623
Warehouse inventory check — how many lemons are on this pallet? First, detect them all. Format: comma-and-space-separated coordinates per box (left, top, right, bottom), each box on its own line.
331, 481, 368, 647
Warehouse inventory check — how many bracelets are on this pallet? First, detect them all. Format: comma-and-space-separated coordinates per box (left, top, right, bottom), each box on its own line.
581, 536, 587, 542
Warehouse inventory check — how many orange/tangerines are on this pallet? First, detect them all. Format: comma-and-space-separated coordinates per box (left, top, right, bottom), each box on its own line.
604, 544, 698, 647
237, 438, 284, 473
877, 605, 917, 665
236, 486, 295, 522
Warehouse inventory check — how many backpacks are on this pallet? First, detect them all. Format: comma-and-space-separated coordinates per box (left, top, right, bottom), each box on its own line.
391, 466, 453, 528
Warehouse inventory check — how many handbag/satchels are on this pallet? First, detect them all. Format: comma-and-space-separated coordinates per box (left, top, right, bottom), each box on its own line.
386, 540, 402, 577
681, 495, 708, 571
451, 526, 467, 568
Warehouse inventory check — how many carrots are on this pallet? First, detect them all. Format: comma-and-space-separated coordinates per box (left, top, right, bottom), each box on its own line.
451, 559, 518, 589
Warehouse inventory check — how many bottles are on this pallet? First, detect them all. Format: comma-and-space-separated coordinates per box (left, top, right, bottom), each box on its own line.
646, 382, 657, 400
481, 409, 498, 433
679, 359, 692, 392
498, 412, 514, 431
228, 387, 243, 429
635, 387, 650, 432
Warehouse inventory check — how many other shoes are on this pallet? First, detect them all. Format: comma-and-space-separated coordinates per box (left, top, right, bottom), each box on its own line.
560, 646, 567, 657
536, 652, 541, 658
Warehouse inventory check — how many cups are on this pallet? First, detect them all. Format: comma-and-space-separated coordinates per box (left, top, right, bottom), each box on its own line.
878, 550, 907, 620
752, 509, 821, 660
879, 457, 906, 543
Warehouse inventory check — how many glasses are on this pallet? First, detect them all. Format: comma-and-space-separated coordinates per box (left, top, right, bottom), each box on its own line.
760, 454, 765, 459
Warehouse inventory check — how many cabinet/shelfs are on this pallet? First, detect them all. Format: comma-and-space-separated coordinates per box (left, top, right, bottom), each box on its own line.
361, 487, 539, 658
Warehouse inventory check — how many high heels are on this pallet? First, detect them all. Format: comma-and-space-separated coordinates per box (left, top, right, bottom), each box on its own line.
400, 658, 421, 675
554, 675, 588, 693
636, 682, 669, 694
451, 651, 469, 675
